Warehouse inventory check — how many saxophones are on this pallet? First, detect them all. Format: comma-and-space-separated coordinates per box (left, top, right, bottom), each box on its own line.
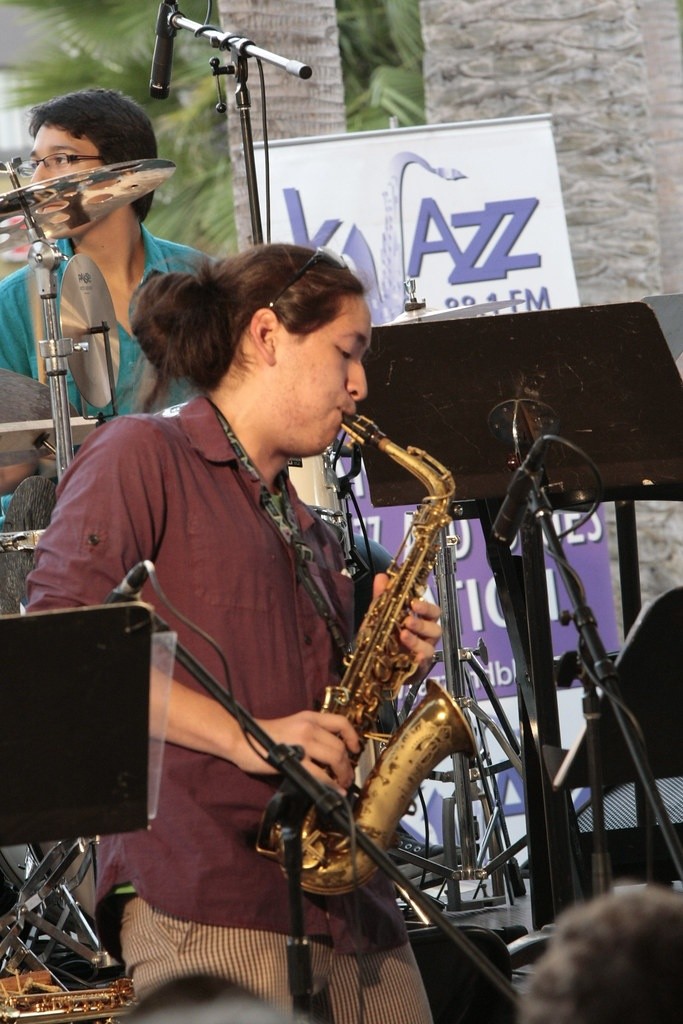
249, 412, 483, 898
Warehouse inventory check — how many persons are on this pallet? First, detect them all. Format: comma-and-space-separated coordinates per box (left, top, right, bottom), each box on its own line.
122, 973, 286, 1024
521, 883, 683, 1024
0, 86, 221, 612
26, 244, 442, 1024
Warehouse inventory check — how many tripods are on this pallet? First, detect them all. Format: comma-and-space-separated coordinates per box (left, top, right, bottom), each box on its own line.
386, 516, 521, 916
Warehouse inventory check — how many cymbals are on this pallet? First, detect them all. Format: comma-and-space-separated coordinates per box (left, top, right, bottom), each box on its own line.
380, 297, 526, 326
1, 368, 80, 470
1, 158, 179, 256
0, 475, 57, 615
59, 254, 120, 409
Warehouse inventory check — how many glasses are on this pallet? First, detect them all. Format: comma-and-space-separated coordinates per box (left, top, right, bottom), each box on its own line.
16, 153, 103, 179
246, 245, 349, 329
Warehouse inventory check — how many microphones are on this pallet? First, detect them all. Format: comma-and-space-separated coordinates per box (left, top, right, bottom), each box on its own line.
103, 563, 148, 606
150, 0, 178, 99
488, 437, 548, 551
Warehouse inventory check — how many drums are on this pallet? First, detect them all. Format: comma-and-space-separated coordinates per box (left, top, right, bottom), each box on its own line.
286, 444, 350, 562
